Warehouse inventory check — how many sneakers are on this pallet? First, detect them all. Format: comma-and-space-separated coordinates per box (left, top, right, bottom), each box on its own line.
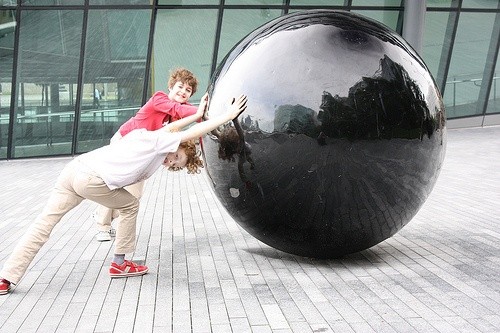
109, 259, 148, 278
96, 232, 111, 241
0, 278, 11, 295
109, 228, 116, 237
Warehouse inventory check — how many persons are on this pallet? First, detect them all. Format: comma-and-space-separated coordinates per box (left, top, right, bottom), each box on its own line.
96, 68, 198, 241
90, 87, 100, 108
0, 93, 249, 294
203, 98, 254, 184
